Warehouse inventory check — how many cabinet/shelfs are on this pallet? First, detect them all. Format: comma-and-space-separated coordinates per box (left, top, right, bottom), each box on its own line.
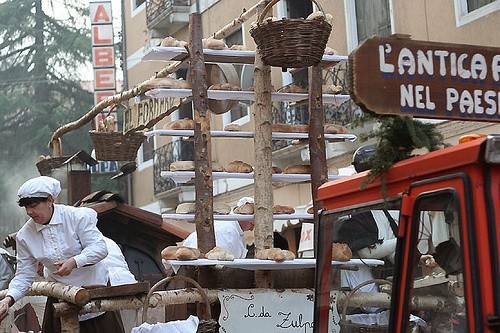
141, 45, 385, 275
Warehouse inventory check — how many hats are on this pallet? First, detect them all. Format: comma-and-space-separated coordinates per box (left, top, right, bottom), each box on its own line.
16, 176, 62, 202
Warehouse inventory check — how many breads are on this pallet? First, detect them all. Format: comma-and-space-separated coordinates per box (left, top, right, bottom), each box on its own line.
224, 160, 253, 173
326, 165, 338, 175
271, 166, 281, 173
323, 125, 347, 134
224, 123, 240, 131
212, 202, 231, 214
248, 17, 277, 28
324, 46, 337, 56
161, 246, 200, 260
256, 247, 296, 261
272, 205, 294, 214
283, 165, 311, 174
270, 79, 309, 94
95, 115, 114, 132
305, 11, 323, 20
144, 77, 188, 89
208, 82, 240, 90
163, 118, 194, 129
321, 84, 342, 95
272, 123, 308, 133
323, 14, 334, 25
176, 202, 196, 214
211, 161, 223, 172
201, 38, 243, 51
331, 243, 352, 262
169, 161, 196, 171
206, 246, 235, 261
160, 36, 187, 48
233, 203, 254, 214
307, 207, 314, 214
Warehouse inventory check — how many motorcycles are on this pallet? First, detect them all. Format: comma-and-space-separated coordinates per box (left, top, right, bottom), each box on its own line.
309, 134, 500, 333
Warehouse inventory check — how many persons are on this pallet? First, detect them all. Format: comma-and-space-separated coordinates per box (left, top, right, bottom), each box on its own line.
172, 196, 255, 288
337, 210, 464, 313
0, 177, 139, 333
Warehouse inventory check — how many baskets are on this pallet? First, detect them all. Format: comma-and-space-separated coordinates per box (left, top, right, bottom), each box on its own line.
37, 139, 86, 190
142, 276, 216, 333
340, 278, 392, 333
88, 105, 147, 162
249, 0, 332, 70
